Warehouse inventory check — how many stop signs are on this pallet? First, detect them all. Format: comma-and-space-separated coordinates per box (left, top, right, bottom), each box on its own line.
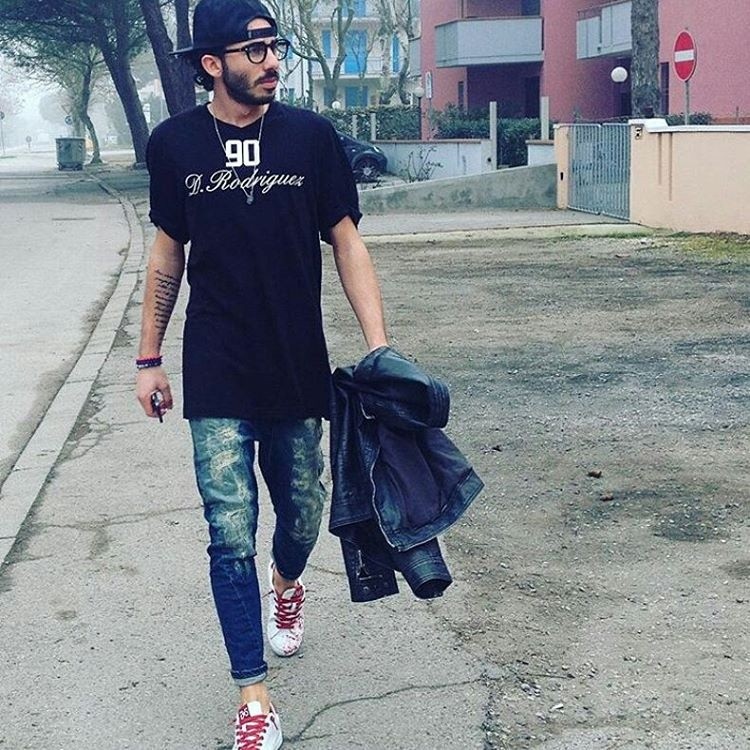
671, 30, 696, 82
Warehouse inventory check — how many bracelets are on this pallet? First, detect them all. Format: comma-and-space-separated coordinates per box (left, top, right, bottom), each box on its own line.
136, 355, 162, 369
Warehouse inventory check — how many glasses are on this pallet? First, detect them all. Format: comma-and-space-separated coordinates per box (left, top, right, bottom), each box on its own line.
201, 38, 290, 64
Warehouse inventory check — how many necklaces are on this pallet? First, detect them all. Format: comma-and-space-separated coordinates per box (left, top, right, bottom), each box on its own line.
212, 99, 265, 204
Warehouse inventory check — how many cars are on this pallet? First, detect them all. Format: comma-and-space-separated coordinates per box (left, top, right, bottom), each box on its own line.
336, 129, 388, 182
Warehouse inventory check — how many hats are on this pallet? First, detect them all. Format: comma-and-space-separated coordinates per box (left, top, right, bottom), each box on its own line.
167, 0, 278, 55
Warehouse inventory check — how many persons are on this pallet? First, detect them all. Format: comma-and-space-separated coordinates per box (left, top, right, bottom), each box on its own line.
136, 0, 388, 750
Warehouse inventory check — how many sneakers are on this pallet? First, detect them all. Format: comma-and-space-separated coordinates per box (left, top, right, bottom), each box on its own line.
232, 701, 283, 750
267, 559, 306, 657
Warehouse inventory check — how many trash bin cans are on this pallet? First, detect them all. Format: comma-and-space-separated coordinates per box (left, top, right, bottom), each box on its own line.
56, 138, 85, 170
